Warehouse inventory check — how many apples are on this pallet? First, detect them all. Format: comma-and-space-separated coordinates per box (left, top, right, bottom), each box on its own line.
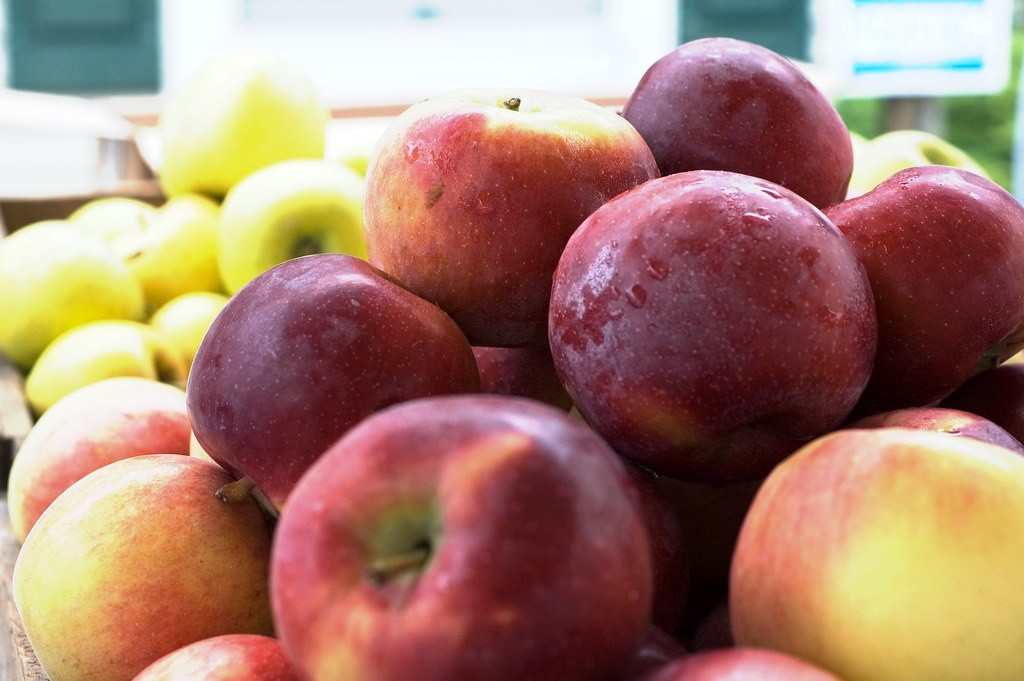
0, 37, 1024, 681
